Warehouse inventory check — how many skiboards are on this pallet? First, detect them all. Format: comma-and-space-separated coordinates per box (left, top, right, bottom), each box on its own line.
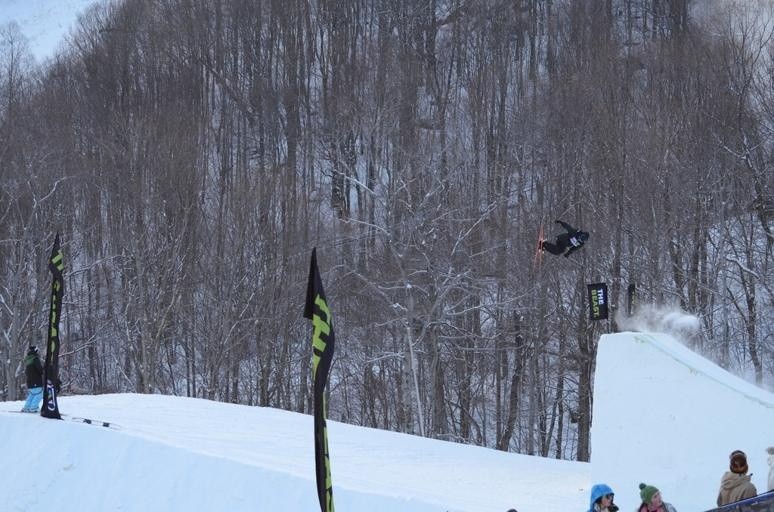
532, 224, 545, 272
8, 411, 64, 415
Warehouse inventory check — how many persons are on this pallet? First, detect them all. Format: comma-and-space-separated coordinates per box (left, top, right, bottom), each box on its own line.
718, 450, 758, 508
538, 218, 589, 259
584, 484, 619, 511
19, 343, 43, 414
635, 481, 677, 512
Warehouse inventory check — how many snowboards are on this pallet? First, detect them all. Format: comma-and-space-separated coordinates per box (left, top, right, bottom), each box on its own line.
72, 416, 120, 428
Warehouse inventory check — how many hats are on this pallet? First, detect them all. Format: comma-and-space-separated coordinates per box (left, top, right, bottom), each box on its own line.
29, 345, 38, 353
638, 483, 660, 501
730, 451, 750, 474
